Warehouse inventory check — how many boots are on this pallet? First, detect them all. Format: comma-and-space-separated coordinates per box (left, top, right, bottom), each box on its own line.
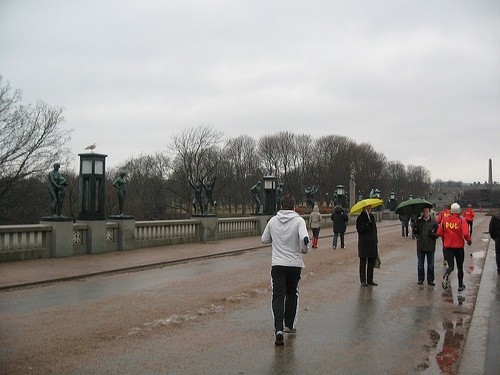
315, 239, 318, 248
312, 239, 315, 248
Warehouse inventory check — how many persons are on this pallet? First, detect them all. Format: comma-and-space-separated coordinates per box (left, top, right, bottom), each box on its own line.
46, 163, 65, 217
113, 172, 127, 216
396, 201, 476, 292
356, 205, 379, 287
333, 190, 348, 207
304, 184, 320, 208
261, 195, 310, 346
309, 205, 322, 249
251, 181, 262, 213
330, 204, 348, 249
187, 178, 216, 215
489, 209, 500, 273
276, 183, 283, 210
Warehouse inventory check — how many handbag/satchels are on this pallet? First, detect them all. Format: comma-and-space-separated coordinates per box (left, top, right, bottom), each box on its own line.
374, 256, 381, 268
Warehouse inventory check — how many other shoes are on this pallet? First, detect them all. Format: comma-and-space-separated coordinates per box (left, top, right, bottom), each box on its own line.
412, 235, 416, 239
361, 282, 367, 286
402, 235, 404, 237
406, 235, 408, 236
418, 280, 423, 284
444, 260, 447, 265
367, 280, 377, 286
428, 281, 435, 286
341, 247, 344, 248
333, 246, 335, 249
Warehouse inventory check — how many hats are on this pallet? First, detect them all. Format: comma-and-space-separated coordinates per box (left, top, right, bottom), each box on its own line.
451, 203, 460, 213
468, 204, 472, 207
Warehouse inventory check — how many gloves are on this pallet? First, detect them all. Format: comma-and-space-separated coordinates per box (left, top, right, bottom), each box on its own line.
467, 241, 472, 246
367, 220, 373, 226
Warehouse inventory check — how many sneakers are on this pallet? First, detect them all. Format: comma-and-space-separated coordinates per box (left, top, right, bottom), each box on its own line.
442, 274, 448, 290
275, 331, 284, 346
458, 285, 466, 291
283, 327, 296, 333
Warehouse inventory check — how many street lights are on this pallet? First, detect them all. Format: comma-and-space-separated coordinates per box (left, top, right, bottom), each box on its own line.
77, 153, 109, 221
263, 176, 278, 215
390, 192, 395, 210
336, 185, 345, 208
375, 190, 382, 211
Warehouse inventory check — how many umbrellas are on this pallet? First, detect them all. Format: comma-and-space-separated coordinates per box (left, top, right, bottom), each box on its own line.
394, 198, 433, 215
349, 197, 383, 215
